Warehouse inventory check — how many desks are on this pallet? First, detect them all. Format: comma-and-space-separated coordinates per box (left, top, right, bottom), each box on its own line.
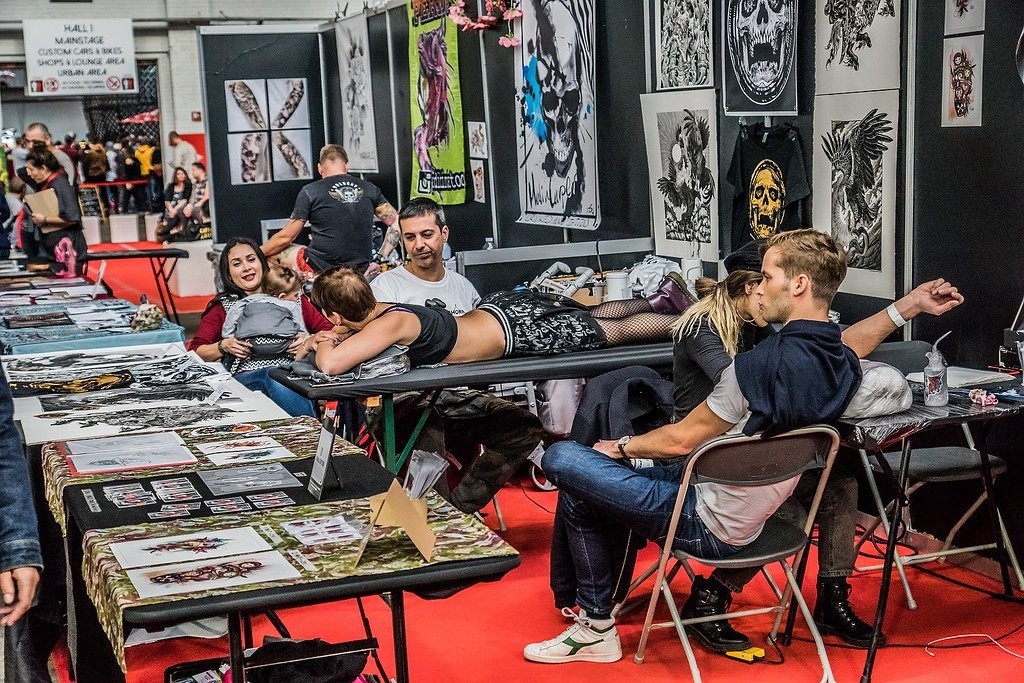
781, 375, 1024, 683
1, 247, 522, 682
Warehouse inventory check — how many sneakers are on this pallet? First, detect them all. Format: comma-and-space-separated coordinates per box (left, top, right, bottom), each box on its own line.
523, 609, 622, 664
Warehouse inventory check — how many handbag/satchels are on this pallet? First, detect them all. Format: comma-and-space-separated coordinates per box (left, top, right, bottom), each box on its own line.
535, 378, 585, 434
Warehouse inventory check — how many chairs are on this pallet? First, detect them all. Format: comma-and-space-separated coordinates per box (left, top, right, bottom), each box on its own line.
849, 341, 1024, 611
583, 365, 792, 618
633, 424, 841, 683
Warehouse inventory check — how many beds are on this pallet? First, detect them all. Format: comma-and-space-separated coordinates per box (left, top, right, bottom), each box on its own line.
268, 338, 675, 477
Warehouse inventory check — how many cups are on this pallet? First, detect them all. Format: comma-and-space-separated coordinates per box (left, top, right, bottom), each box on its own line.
681, 259, 704, 281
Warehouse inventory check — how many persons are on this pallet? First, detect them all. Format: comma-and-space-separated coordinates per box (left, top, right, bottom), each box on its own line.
220, 265, 313, 373
363, 196, 540, 525
522, 226, 964, 665
191, 233, 336, 419
674, 238, 888, 650
258, 143, 401, 277
290, 266, 700, 377
0, 124, 211, 272
1, 359, 44, 625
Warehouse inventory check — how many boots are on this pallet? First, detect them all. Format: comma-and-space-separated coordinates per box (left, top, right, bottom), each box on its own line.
812, 575, 886, 647
647, 271, 700, 313
677, 575, 752, 651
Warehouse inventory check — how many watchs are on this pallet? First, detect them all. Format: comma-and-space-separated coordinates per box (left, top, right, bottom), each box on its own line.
617, 436, 632, 460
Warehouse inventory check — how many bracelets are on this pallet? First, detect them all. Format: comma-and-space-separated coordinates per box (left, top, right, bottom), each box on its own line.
887, 301, 906, 327
217, 340, 227, 355
303, 336, 310, 353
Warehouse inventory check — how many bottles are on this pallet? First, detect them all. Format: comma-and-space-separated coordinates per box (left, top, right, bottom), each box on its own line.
924, 351, 949, 407
481, 237, 498, 250
605, 271, 628, 302
